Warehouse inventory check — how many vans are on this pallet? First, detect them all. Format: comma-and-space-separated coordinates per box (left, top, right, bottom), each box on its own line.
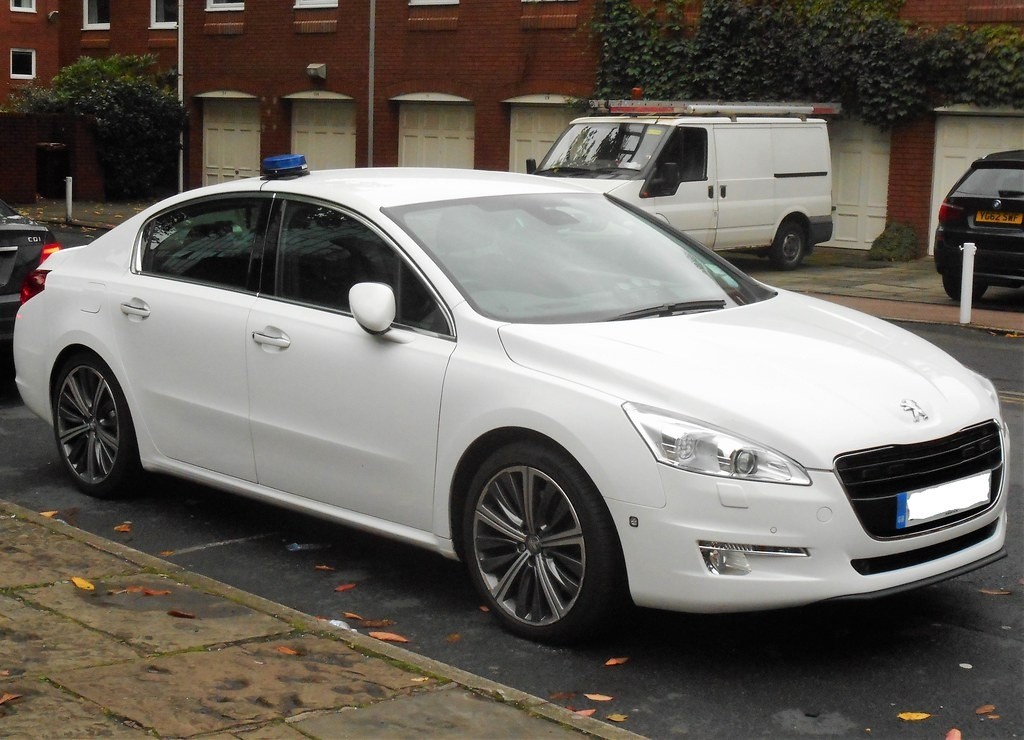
524, 88, 843, 271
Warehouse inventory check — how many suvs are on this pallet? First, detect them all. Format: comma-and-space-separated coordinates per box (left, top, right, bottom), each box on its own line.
934, 149, 1024, 300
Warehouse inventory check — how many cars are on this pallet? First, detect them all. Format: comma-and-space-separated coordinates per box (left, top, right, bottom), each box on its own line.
0, 195, 63, 400
14, 154, 1007, 647
